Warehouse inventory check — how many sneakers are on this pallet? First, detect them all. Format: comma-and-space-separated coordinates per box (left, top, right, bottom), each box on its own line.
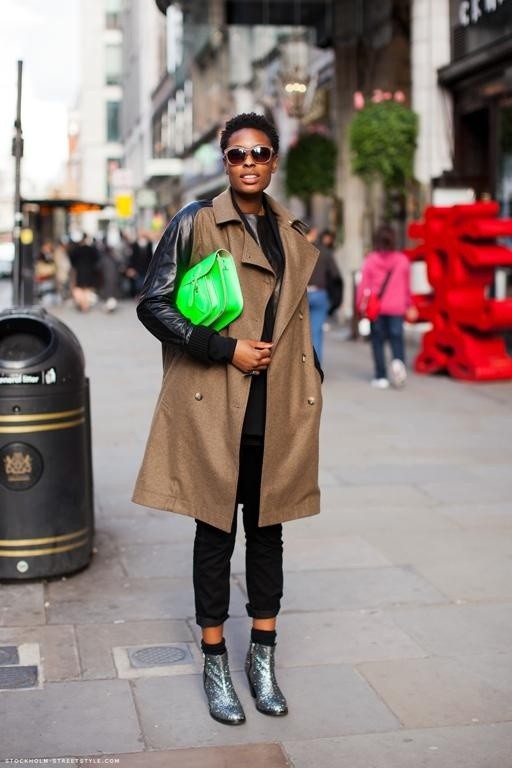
369, 377, 390, 391
391, 358, 408, 388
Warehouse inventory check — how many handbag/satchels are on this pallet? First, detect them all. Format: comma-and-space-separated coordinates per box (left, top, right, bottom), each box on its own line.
364, 290, 382, 321
175, 249, 244, 332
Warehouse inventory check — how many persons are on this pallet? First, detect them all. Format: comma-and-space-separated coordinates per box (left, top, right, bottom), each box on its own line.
39, 231, 153, 312
130, 111, 326, 726
354, 226, 411, 389
304, 225, 344, 367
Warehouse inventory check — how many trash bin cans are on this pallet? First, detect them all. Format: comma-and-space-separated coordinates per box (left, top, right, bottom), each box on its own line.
0, 307, 93, 579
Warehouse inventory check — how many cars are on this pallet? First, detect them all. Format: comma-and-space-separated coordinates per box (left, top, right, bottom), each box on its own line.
0, 242, 16, 278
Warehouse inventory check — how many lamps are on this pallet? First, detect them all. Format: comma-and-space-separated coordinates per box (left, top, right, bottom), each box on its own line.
274, 70, 312, 118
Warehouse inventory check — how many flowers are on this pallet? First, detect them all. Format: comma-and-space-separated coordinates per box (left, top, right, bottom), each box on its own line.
347, 88, 418, 222
283, 124, 338, 202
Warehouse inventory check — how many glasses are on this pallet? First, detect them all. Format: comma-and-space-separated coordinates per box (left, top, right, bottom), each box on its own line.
222, 143, 276, 166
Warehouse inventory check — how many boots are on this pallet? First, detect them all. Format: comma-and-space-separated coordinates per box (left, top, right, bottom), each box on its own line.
202, 650, 246, 726
245, 641, 288, 716
